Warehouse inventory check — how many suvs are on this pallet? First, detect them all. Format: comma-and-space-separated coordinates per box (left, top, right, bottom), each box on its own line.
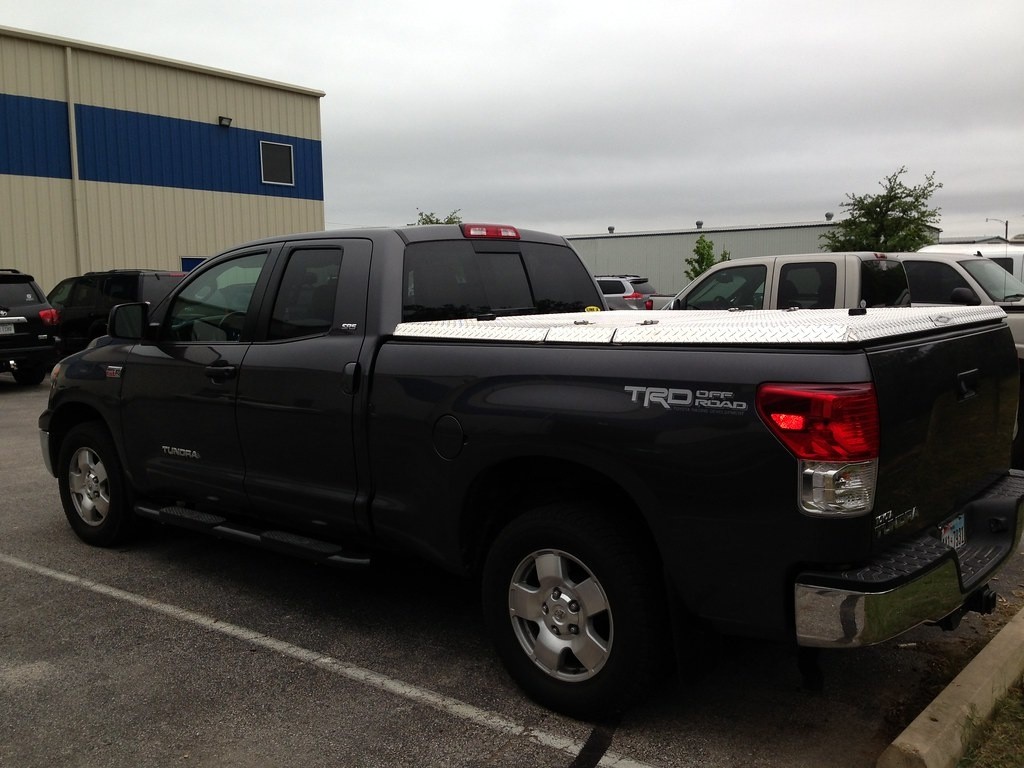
44, 267, 198, 360
592, 274, 657, 311
0, 268, 66, 386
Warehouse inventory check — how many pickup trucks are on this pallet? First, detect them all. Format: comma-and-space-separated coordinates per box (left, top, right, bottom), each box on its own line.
37, 221, 1024, 721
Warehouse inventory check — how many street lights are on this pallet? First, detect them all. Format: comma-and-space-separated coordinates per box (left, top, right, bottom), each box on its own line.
986, 218, 1008, 239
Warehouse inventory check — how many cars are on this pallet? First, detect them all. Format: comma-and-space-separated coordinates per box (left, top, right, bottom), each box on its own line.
660, 239, 1024, 383
